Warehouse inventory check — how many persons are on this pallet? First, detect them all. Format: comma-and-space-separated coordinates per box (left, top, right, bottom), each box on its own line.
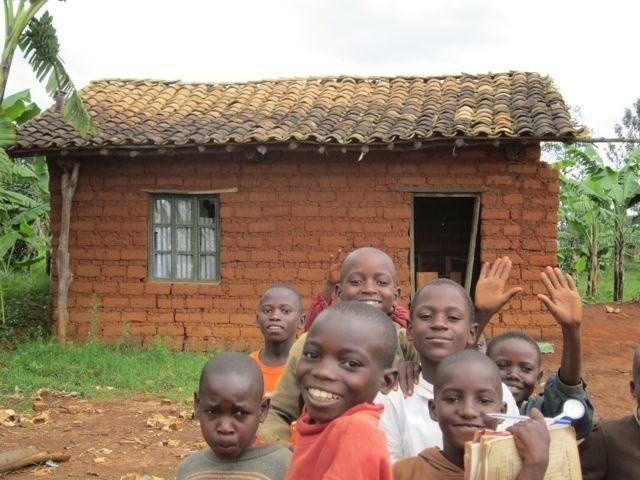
249, 284, 306, 399
304, 253, 410, 331
177, 352, 293, 480
578, 347, 640, 479
257, 248, 421, 444
474, 256, 595, 441
285, 301, 399, 480
391, 349, 550, 480
373, 278, 521, 466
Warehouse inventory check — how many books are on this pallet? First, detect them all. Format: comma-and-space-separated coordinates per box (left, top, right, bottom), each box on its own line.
464, 424, 582, 480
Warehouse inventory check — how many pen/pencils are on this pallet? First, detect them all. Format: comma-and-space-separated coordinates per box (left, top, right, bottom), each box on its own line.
485, 413, 571, 425
